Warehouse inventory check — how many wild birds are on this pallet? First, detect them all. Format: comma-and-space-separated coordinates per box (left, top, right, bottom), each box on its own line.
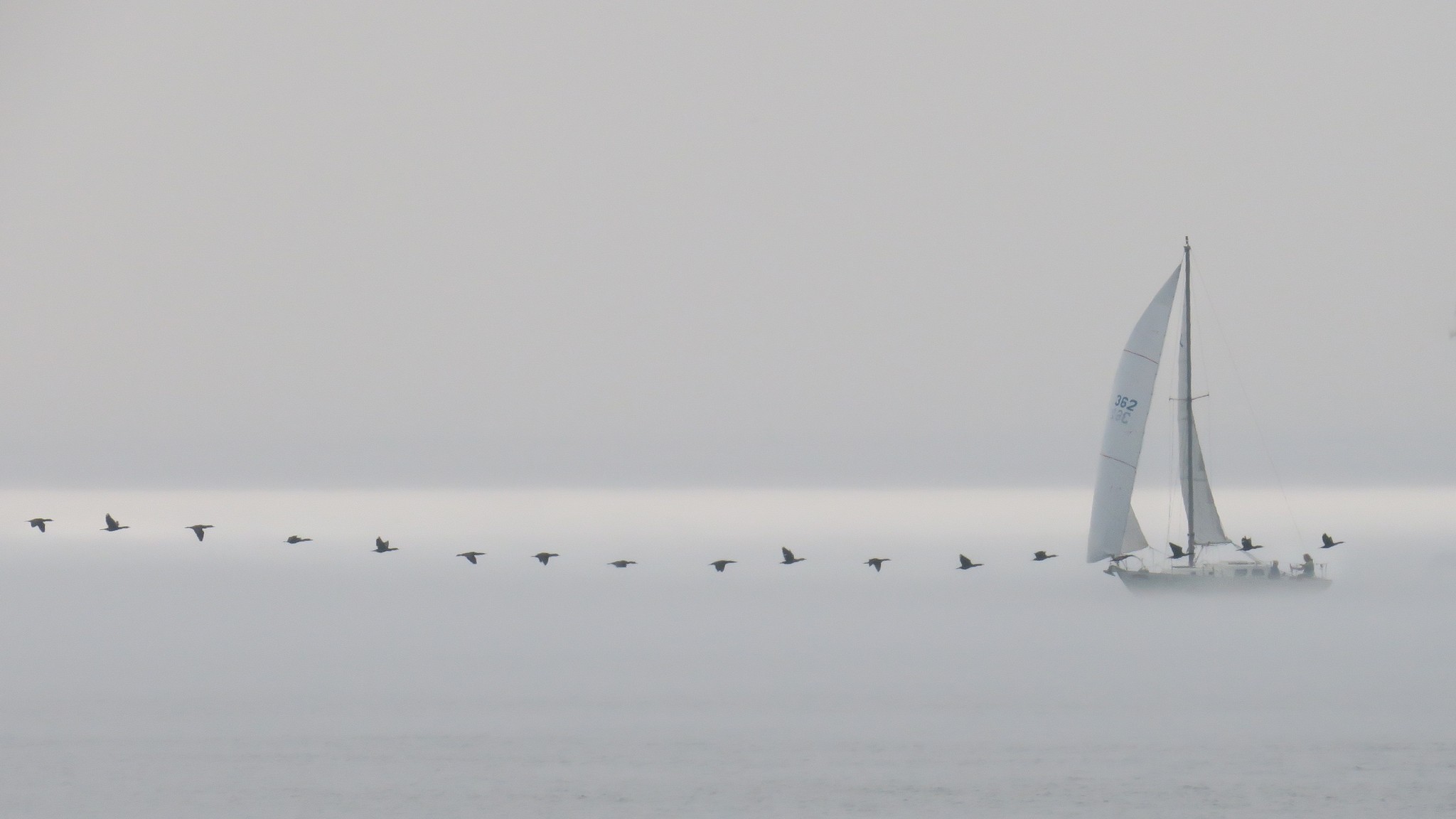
184, 525, 215, 541
456, 551, 486, 564
1109, 555, 1133, 561
957, 554, 984, 569
608, 560, 637, 568
1235, 536, 1264, 551
99, 513, 131, 531
1319, 534, 1345, 549
778, 547, 807, 564
284, 536, 314, 544
371, 537, 399, 552
864, 558, 891, 572
709, 560, 737, 572
532, 552, 560, 566
1168, 542, 1195, 560
1031, 551, 1058, 561
24, 518, 53, 533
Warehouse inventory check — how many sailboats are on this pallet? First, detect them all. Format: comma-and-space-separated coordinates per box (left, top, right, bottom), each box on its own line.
1085, 235, 1337, 594
